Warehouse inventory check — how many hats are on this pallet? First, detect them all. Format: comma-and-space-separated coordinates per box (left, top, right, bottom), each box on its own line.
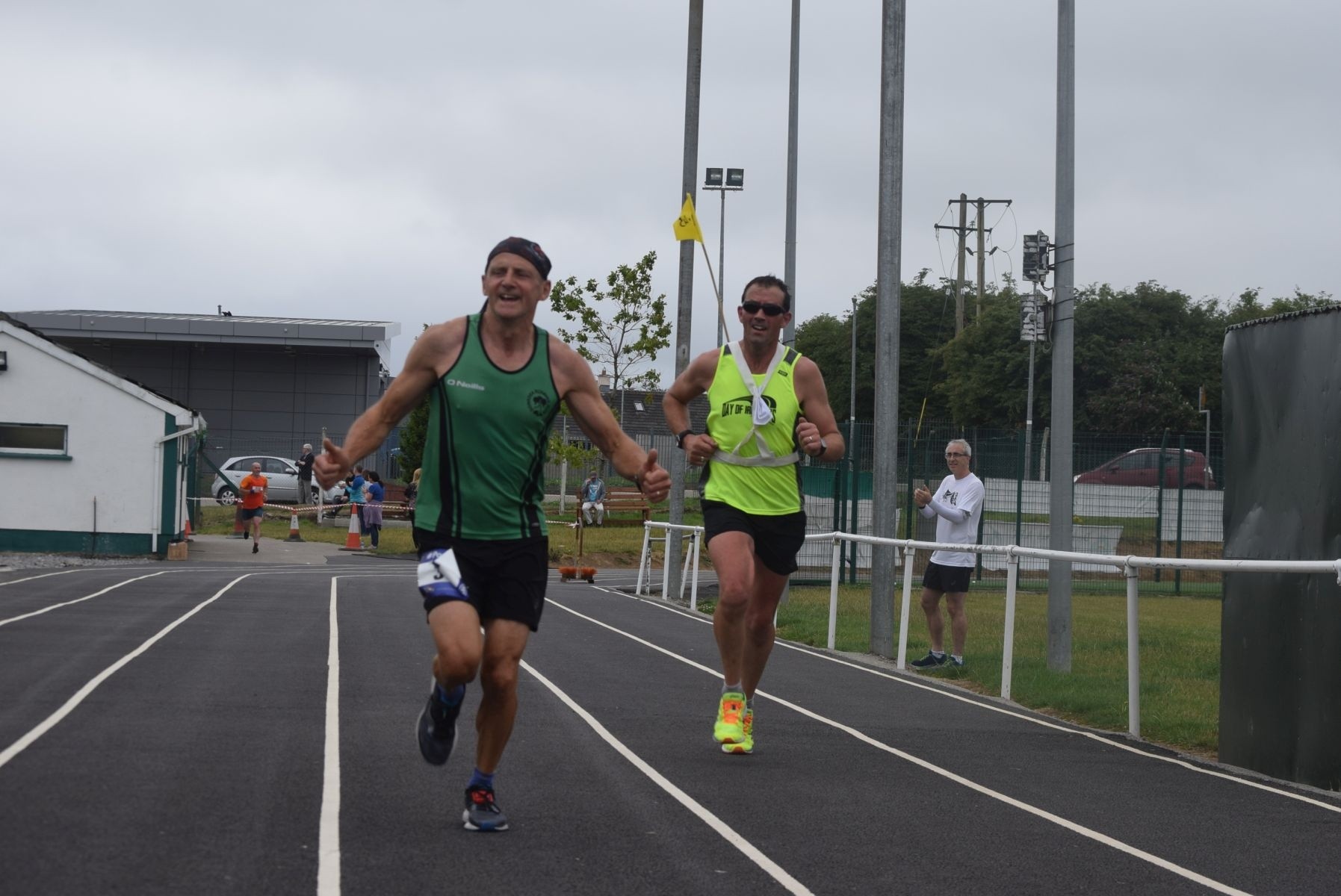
485, 237, 552, 280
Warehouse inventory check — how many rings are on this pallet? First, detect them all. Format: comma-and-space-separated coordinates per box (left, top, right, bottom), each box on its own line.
657, 491, 660, 496
809, 436, 813, 442
701, 456, 704, 462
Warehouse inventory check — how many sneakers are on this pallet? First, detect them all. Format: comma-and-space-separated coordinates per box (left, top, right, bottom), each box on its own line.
712, 690, 746, 743
722, 708, 754, 754
415, 675, 466, 767
910, 650, 947, 668
462, 781, 508, 832
938, 656, 963, 669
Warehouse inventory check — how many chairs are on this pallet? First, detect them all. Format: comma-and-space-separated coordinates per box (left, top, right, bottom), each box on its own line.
1117, 458, 1145, 470
266, 464, 281, 473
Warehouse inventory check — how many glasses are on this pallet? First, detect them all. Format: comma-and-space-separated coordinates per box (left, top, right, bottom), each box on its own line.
945, 452, 968, 459
742, 301, 785, 318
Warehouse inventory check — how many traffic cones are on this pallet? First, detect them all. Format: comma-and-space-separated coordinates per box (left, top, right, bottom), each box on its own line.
337, 502, 370, 551
283, 506, 306, 542
225, 500, 251, 539
185, 502, 196, 536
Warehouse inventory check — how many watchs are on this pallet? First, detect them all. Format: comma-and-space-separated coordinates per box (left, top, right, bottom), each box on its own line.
815, 439, 827, 456
675, 430, 694, 449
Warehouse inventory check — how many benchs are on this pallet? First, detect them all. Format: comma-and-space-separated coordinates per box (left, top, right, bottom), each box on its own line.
377, 482, 410, 520
576, 486, 653, 527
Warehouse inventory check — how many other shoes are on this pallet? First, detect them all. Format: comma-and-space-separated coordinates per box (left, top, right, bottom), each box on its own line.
244, 532, 249, 539
252, 542, 259, 553
367, 545, 378, 549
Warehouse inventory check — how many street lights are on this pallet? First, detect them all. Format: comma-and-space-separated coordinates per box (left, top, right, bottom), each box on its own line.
701, 166, 745, 348
965, 245, 1000, 325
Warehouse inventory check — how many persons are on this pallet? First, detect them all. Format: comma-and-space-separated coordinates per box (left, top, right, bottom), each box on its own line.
663, 275, 845, 755
403, 468, 421, 547
238, 461, 269, 554
580, 470, 605, 526
326, 465, 385, 549
910, 439, 986, 669
311, 236, 671, 834
295, 444, 315, 504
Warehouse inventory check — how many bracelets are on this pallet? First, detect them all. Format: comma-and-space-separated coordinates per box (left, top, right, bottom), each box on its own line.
634, 473, 642, 494
251, 490, 254, 493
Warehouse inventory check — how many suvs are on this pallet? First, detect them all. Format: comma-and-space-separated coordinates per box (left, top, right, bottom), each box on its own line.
211, 456, 347, 508
1073, 447, 1215, 491
